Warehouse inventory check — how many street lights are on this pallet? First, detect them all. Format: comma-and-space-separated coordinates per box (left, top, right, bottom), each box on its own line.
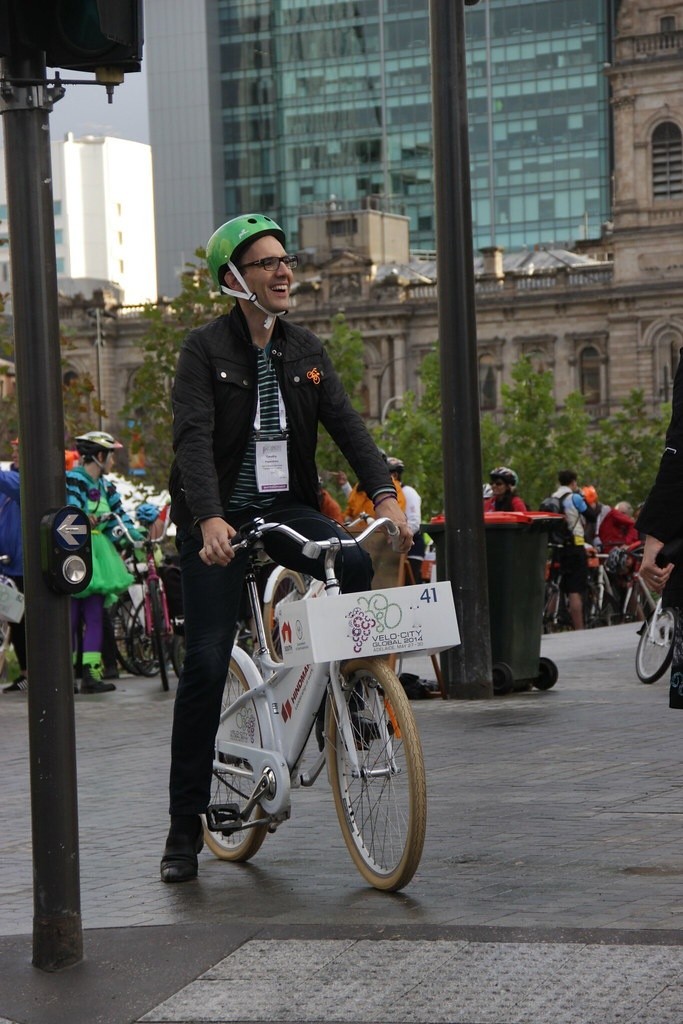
376, 346, 437, 427
87, 310, 117, 432
381, 395, 404, 423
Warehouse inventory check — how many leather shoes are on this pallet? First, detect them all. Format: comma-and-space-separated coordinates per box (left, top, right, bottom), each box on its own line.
160, 814, 204, 882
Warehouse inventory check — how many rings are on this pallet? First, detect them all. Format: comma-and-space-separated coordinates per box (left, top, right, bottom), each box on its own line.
653, 575, 658, 579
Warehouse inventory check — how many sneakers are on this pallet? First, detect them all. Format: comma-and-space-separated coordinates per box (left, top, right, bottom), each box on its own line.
79, 663, 117, 694
2, 675, 30, 695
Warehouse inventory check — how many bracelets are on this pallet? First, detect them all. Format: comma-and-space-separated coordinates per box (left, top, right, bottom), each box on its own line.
373, 495, 397, 511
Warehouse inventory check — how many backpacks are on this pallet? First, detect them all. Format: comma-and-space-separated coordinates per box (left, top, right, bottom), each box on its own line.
538, 492, 573, 545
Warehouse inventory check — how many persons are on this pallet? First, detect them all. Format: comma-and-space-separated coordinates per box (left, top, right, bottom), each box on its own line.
318, 446, 423, 535
545, 471, 651, 631
58, 431, 174, 695
0, 435, 28, 693
634, 345, 683, 710
160, 211, 416, 884
482, 467, 528, 512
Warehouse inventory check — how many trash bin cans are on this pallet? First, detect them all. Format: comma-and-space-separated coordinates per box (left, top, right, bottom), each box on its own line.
416, 511, 567, 699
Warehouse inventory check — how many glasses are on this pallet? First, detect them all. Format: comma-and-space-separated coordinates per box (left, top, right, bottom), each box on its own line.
238, 253, 300, 272
488, 479, 504, 487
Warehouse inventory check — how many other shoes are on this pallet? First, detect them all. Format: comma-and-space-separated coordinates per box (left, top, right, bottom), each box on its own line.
314, 721, 371, 754
99, 667, 120, 680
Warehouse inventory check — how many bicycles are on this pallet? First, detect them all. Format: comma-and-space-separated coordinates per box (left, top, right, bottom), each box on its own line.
0, 555, 17, 671
198, 512, 428, 892
635, 596, 676, 685
98, 505, 185, 693
542, 543, 660, 636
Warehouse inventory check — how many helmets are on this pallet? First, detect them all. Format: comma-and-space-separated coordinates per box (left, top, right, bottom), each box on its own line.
205, 214, 286, 298
386, 457, 404, 472
378, 447, 388, 461
491, 466, 519, 492
74, 430, 124, 456
135, 503, 160, 522
482, 483, 493, 499
317, 475, 324, 487
581, 486, 598, 505
